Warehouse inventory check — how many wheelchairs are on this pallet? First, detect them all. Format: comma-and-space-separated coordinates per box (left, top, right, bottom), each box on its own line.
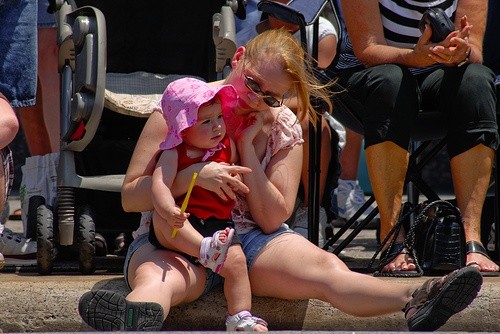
36, 0, 245, 275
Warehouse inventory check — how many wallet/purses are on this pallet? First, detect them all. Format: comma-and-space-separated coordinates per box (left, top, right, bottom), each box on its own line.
418, 8, 455, 43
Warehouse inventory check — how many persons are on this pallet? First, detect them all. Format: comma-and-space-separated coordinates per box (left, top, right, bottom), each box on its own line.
230, 0, 500, 277
152, 77, 268, 332
77, 30, 484, 332
0, 0, 59, 272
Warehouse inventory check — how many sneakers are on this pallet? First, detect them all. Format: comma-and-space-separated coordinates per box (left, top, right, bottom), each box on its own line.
290, 203, 327, 248
330, 180, 380, 222
0, 225, 58, 266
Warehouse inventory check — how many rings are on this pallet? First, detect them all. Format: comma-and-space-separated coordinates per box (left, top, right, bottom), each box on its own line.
448, 55, 453, 62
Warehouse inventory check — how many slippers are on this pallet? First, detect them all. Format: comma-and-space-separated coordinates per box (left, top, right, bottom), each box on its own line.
466, 240, 500, 276
379, 243, 418, 273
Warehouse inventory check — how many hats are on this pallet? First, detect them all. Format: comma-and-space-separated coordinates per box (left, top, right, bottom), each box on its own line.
159, 77, 240, 150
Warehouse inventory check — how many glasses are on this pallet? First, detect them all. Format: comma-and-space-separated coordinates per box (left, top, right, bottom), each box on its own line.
243, 47, 284, 108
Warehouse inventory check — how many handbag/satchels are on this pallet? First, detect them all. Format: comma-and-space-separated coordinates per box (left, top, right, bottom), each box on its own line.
368, 199, 466, 277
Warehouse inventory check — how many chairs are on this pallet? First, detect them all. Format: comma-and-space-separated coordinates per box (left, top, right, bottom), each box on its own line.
257, 0, 500, 267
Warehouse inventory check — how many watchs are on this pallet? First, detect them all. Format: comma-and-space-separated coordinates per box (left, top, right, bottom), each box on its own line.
458, 47, 471, 67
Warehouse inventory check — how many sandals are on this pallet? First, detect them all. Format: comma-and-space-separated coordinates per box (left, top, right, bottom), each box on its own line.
78, 290, 164, 331
401, 267, 483, 332
226, 310, 268, 332
195, 229, 235, 274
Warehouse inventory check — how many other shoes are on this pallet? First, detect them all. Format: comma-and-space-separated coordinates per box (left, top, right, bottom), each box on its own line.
113, 233, 125, 255
95, 233, 107, 257
